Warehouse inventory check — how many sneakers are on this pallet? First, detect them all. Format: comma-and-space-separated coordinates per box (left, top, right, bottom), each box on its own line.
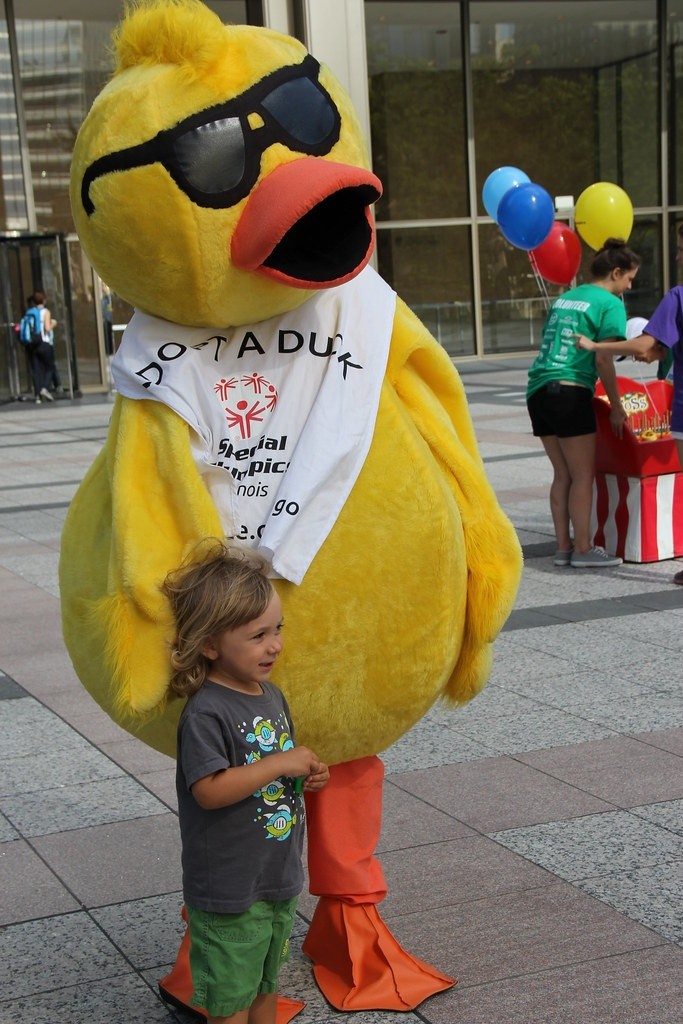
554, 548, 575, 566
571, 545, 623, 567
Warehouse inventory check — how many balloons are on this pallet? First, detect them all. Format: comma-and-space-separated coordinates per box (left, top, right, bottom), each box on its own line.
574, 181, 634, 253
527, 223, 582, 285
482, 166, 554, 250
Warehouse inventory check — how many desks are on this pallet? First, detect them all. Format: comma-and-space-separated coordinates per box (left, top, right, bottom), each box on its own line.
589, 472, 683, 564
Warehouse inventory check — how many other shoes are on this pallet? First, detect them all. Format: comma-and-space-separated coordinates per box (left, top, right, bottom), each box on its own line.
36, 396, 42, 403
674, 571, 683, 584
40, 387, 54, 402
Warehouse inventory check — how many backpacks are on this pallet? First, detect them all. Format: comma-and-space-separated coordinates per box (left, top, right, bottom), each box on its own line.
19, 306, 47, 344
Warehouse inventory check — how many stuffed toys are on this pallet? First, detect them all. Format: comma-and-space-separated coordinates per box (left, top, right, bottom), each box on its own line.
58, 3, 524, 1024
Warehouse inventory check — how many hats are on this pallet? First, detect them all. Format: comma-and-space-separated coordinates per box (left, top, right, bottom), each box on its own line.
615, 316, 650, 362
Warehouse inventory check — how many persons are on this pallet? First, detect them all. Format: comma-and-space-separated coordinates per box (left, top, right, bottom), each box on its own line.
176, 556, 330, 1024
525, 239, 641, 568
28, 293, 57, 404
568, 222, 683, 585
